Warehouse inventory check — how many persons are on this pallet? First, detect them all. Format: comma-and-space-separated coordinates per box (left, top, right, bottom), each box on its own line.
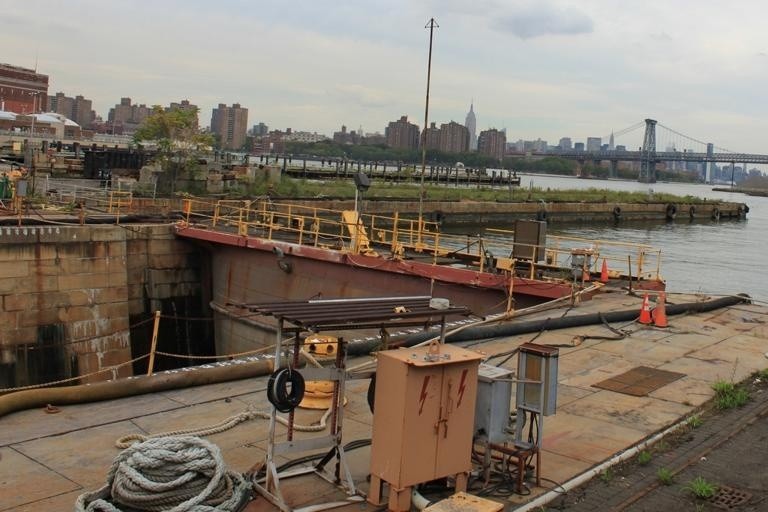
100, 167, 106, 188
107, 167, 112, 188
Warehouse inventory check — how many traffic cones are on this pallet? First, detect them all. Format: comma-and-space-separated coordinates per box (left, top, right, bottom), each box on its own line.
600, 258, 608, 283
655, 292, 667, 327
637, 291, 653, 324
